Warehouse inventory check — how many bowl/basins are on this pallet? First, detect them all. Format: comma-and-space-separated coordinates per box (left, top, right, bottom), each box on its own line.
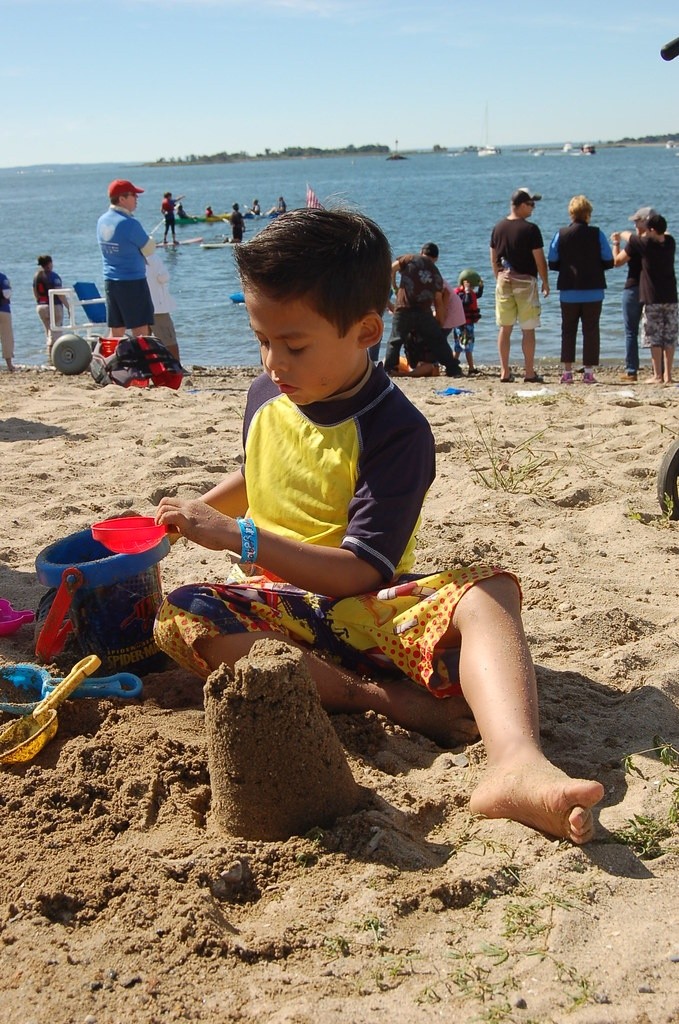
91, 517, 166, 553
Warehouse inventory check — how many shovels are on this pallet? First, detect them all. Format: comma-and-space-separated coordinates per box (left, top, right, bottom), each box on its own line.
0, 655, 102, 762
0, 662, 143, 714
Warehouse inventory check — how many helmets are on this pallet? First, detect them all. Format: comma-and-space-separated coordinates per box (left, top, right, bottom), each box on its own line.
458, 271, 481, 286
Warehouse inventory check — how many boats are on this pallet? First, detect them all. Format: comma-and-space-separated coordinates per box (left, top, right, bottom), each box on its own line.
386, 155, 407, 161
199, 243, 246, 248
447, 145, 501, 157
164, 212, 287, 225
528, 144, 596, 156
665, 141, 676, 149
154, 237, 204, 248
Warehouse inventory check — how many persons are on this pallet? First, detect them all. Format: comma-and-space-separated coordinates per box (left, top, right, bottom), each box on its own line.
154, 204, 604, 846
1, 181, 679, 384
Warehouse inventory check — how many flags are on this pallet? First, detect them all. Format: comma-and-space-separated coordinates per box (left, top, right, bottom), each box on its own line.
306, 188, 325, 209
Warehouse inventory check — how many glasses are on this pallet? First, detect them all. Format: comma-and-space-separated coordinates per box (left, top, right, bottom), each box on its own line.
524, 201, 534, 206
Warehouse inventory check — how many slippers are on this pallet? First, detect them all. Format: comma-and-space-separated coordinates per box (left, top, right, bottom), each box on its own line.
500, 374, 515, 383
524, 372, 544, 382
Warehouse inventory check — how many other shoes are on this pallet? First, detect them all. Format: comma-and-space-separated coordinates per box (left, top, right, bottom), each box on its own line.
560, 372, 574, 383
469, 369, 485, 376
582, 373, 597, 382
620, 373, 638, 381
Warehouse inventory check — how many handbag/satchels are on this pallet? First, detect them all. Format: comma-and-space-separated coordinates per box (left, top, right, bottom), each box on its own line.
90, 334, 184, 389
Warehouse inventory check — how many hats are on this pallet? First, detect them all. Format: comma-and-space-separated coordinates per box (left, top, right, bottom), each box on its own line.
512, 187, 542, 203
109, 180, 145, 196
628, 207, 656, 221
422, 243, 440, 256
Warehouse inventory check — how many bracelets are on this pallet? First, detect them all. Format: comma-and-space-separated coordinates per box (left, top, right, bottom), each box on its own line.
234, 517, 258, 564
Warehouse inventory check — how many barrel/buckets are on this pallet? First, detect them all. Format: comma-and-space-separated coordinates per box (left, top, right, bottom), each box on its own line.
35, 526, 172, 670
